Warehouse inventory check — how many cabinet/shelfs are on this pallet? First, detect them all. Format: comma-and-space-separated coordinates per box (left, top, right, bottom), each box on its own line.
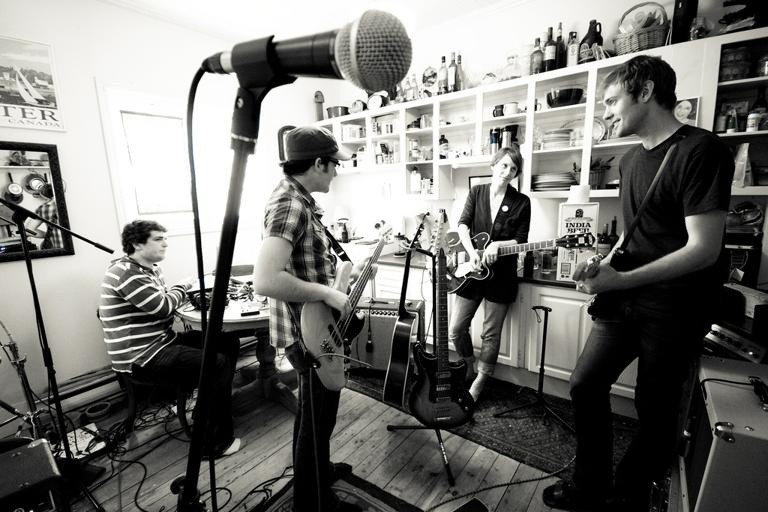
312, 27, 768, 418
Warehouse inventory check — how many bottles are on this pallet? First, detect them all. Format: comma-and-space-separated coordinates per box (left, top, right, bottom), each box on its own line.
396, 49, 464, 100
329, 224, 336, 239
501, 125, 512, 148
523, 250, 535, 276
348, 111, 475, 195
342, 223, 349, 243
607, 216, 620, 250
712, 109, 762, 134
596, 223, 611, 258
489, 127, 500, 155
494, 20, 579, 81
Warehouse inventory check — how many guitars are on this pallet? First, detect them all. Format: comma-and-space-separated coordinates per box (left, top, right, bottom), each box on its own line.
573, 246, 625, 314
405, 210, 476, 428
297, 226, 394, 391
430, 228, 596, 295
382, 212, 431, 411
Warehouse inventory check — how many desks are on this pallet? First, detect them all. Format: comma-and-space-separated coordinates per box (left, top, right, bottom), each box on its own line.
174, 285, 299, 415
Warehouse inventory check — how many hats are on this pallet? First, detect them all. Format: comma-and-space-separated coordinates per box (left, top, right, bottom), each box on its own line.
286, 126, 351, 162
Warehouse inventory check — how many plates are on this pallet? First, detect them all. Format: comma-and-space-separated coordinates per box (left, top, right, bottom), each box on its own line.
542, 128, 572, 150
531, 171, 575, 191
559, 117, 605, 145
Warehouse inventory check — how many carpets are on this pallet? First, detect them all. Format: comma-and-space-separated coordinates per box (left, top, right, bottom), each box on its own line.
342, 369, 638, 480
257, 473, 424, 512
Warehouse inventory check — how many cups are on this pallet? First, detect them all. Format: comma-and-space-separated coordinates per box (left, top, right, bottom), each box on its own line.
540, 250, 552, 275
492, 101, 518, 117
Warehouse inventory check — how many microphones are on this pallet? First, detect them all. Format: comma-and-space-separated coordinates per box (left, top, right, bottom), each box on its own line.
204, 8, 412, 92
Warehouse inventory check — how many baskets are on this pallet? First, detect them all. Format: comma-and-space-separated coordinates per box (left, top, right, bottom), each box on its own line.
612, 2, 671, 56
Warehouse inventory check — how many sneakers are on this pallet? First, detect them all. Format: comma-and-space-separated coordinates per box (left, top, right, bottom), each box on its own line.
543, 477, 614, 509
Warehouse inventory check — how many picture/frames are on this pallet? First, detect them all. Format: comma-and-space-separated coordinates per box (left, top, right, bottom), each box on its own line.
0, 141, 75, 263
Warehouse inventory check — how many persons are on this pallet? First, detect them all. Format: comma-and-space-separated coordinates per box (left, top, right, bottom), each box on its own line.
542, 54, 734, 512
251, 124, 378, 512
24, 184, 63, 249
448, 148, 532, 406
98, 222, 245, 461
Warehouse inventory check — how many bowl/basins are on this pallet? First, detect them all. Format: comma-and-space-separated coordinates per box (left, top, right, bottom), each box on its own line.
571, 170, 608, 188
546, 88, 584, 108
326, 89, 388, 117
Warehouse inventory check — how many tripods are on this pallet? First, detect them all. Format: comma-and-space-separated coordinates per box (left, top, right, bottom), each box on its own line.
0, 195, 116, 512
385, 255, 473, 494
493, 306, 577, 435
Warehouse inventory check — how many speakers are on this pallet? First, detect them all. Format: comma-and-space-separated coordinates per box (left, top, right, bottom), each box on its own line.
0, 438, 62, 507
699, 282, 768, 364
348, 297, 425, 378
